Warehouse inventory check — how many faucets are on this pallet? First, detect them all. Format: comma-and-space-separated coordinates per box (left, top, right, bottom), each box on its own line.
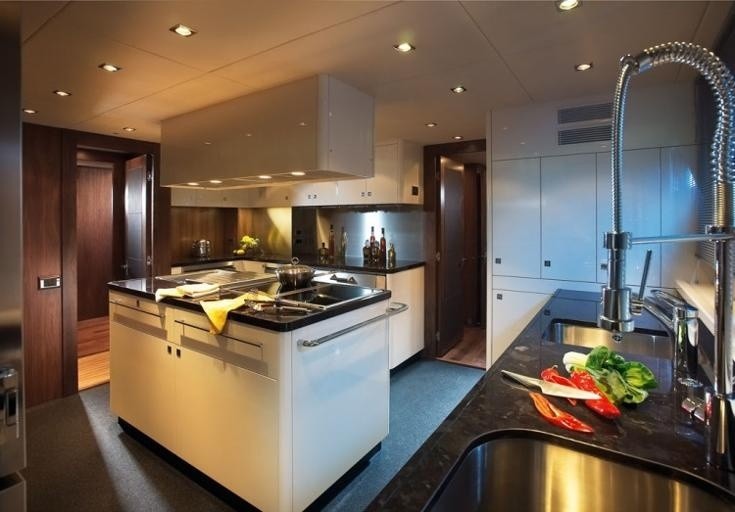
597, 41, 734, 465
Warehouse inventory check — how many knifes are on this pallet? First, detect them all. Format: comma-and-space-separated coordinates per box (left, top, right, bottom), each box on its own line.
502, 368, 605, 400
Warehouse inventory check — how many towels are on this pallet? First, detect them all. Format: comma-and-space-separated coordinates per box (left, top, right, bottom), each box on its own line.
198, 292, 275, 336
152, 284, 220, 303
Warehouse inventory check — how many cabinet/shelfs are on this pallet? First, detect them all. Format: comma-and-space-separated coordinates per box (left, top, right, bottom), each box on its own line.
491, 289, 553, 365
491, 154, 597, 283
171, 187, 248, 207
248, 184, 289, 208
595, 150, 704, 285
289, 182, 338, 206
339, 138, 422, 207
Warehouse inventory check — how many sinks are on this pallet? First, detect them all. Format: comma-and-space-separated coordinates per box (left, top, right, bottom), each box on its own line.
423, 428, 735, 512
546, 318, 675, 362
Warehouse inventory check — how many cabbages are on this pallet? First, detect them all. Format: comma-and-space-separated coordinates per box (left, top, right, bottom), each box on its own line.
564, 346, 658, 404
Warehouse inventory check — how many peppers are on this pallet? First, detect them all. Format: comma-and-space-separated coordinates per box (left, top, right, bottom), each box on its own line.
570, 370, 622, 420
529, 391, 593, 434
540, 365, 579, 408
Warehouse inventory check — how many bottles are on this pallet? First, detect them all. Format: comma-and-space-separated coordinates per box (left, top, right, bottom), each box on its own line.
364, 240, 370, 268
339, 226, 346, 266
370, 226, 375, 266
374, 243, 382, 265
381, 228, 387, 265
318, 242, 329, 265
389, 244, 395, 268
328, 225, 335, 265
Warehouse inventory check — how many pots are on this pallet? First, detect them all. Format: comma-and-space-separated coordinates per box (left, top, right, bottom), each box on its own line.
193, 239, 212, 258
274, 258, 317, 287
